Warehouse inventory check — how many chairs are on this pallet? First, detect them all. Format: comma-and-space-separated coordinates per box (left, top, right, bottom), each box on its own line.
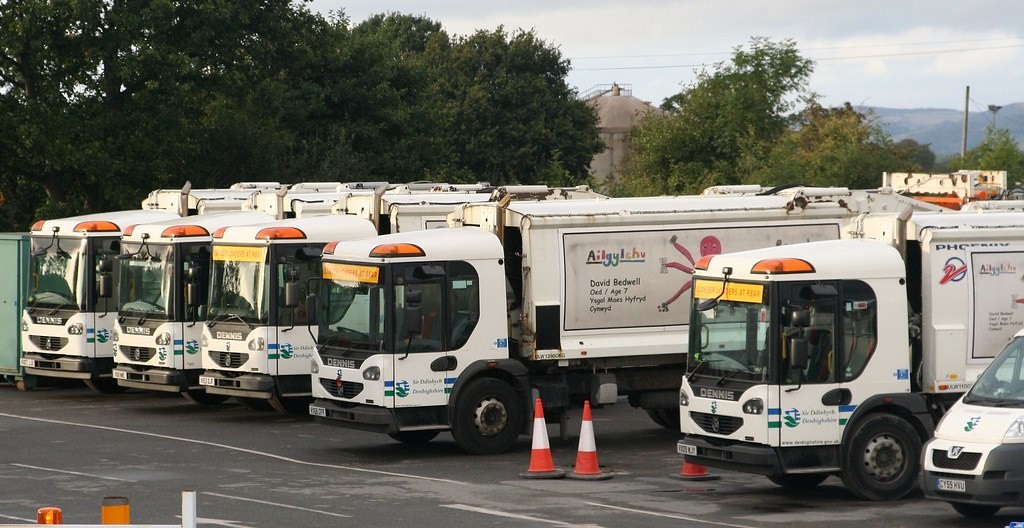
405, 291, 424, 333
800, 329, 833, 382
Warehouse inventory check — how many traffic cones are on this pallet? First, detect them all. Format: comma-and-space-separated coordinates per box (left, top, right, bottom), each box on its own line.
669, 458, 720, 482
565, 400, 612, 480
519, 397, 564, 479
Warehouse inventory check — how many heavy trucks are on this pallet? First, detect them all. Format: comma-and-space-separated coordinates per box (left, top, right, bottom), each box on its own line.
14, 186, 227, 394
302, 190, 870, 453
673, 206, 1023, 502
201, 178, 613, 419
107, 179, 344, 406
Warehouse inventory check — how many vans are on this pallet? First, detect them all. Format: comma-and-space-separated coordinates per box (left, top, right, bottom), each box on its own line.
920, 329, 1023, 520
